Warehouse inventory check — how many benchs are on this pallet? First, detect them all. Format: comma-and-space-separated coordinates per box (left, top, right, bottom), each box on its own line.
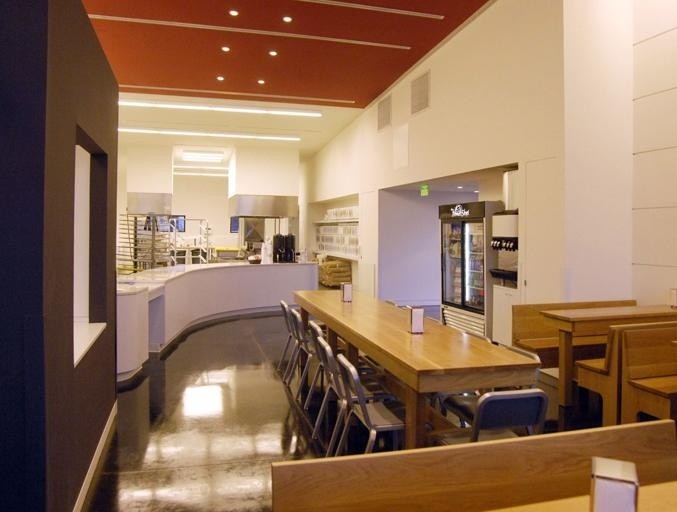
511, 298, 676, 426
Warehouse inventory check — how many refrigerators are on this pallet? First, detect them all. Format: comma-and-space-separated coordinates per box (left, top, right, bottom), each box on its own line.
438, 200, 505, 314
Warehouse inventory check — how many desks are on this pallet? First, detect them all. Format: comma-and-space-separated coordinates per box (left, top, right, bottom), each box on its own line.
291, 289, 542, 449
